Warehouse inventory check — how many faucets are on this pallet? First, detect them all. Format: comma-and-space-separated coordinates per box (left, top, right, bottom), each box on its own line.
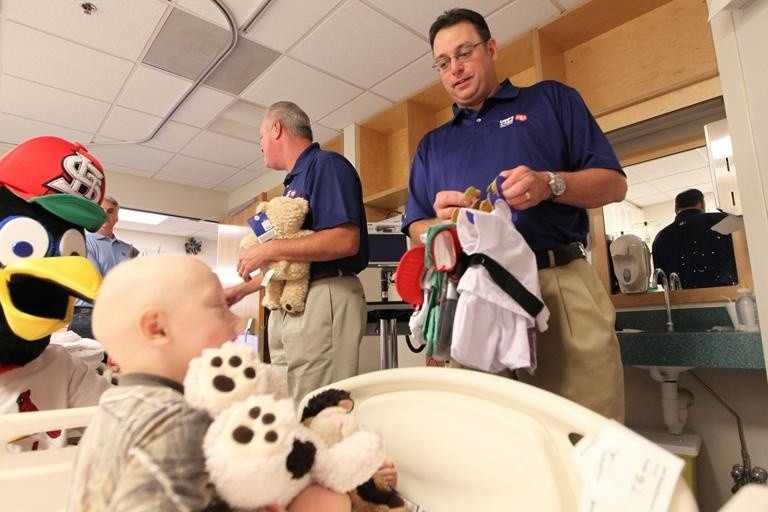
648, 267, 675, 331
669, 272, 682, 291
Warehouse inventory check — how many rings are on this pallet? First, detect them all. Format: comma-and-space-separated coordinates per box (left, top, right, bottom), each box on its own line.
525, 191, 532, 203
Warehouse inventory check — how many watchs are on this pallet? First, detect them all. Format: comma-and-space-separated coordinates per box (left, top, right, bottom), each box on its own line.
543, 168, 564, 203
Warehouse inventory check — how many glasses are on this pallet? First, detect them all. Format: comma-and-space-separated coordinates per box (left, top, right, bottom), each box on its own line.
432, 41, 486, 72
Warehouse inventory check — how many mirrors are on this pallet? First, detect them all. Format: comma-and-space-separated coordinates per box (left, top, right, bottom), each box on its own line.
589, 134, 755, 308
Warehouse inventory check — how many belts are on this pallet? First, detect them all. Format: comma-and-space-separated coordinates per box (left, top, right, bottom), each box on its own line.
534, 243, 586, 270
310, 269, 355, 281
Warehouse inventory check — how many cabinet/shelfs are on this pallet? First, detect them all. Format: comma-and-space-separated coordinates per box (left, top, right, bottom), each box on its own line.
356, 0, 723, 223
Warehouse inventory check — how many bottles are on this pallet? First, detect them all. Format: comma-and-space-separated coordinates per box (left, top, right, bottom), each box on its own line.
735, 287, 759, 328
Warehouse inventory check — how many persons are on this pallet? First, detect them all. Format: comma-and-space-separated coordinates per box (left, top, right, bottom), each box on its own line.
66, 196, 139, 374
651, 189, 737, 289
399, 10, 626, 426
221, 101, 370, 407
65, 252, 398, 512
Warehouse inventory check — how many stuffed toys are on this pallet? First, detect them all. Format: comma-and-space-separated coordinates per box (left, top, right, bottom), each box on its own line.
237, 196, 311, 315
0, 135, 119, 456
181, 340, 411, 512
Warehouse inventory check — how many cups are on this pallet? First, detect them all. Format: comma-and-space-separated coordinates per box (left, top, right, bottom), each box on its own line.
727, 302, 743, 331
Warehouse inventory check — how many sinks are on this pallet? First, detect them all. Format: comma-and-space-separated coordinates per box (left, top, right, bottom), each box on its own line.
614, 329, 766, 374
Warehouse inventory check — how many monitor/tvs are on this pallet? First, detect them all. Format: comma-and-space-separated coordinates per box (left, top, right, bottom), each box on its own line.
365, 232, 410, 268
